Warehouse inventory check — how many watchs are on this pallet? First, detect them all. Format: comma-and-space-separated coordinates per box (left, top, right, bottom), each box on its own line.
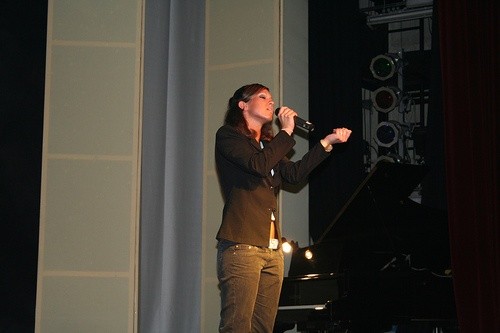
320, 138, 333, 151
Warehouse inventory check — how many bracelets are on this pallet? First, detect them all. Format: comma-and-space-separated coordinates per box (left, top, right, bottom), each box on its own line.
281, 128, 293, 132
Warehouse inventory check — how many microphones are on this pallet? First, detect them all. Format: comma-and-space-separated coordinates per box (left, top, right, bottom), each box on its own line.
275, 108, 314, 132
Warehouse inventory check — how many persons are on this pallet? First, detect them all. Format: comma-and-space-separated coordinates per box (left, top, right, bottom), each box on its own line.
214, 83, 352, 333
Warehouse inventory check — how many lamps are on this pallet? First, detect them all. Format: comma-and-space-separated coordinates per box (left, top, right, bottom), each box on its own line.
370, 49, 412, 147
